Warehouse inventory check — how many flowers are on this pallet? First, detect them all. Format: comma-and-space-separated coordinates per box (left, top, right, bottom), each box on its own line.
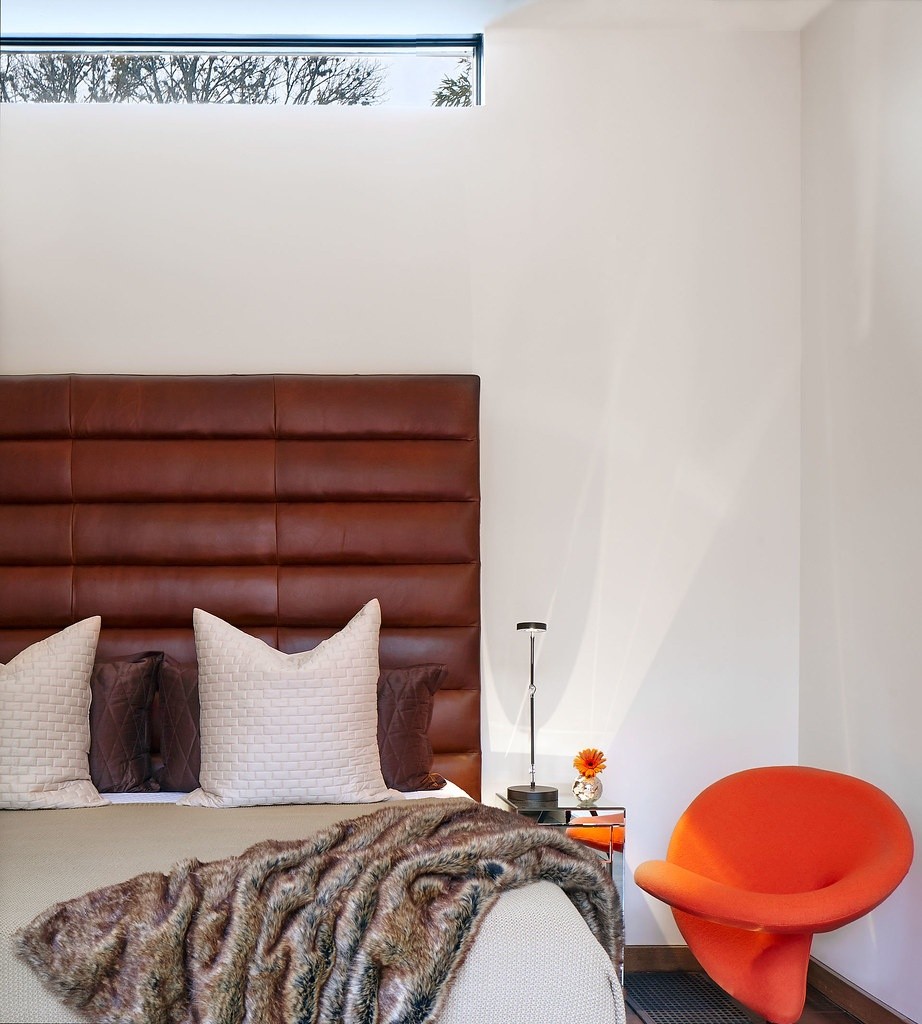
572, 747, 609, 777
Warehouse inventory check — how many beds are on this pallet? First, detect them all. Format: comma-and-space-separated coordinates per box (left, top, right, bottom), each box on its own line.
1, 369, 625, 1024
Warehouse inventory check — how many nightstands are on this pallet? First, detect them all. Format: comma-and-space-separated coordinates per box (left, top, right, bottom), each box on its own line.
496, 795, 625, 880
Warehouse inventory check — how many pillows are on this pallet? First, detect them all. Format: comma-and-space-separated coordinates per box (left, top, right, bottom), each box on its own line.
1, 616, 105, 811
88, 650, 166, 793
176, 597, 405, 809
161, 653, 446, 793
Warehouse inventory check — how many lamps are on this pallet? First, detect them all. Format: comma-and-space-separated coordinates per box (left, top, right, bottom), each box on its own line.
508, 620, 559, 814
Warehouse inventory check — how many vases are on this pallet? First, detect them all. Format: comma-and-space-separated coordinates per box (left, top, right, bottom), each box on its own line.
573, 773, 604, 807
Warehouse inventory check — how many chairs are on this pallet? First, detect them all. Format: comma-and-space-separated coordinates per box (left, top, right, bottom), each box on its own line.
635, 765, 916, 1022
564, 812, 625, 855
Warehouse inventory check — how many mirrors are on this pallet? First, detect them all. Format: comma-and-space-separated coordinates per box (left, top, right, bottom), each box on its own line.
544, 810, 624, 912
